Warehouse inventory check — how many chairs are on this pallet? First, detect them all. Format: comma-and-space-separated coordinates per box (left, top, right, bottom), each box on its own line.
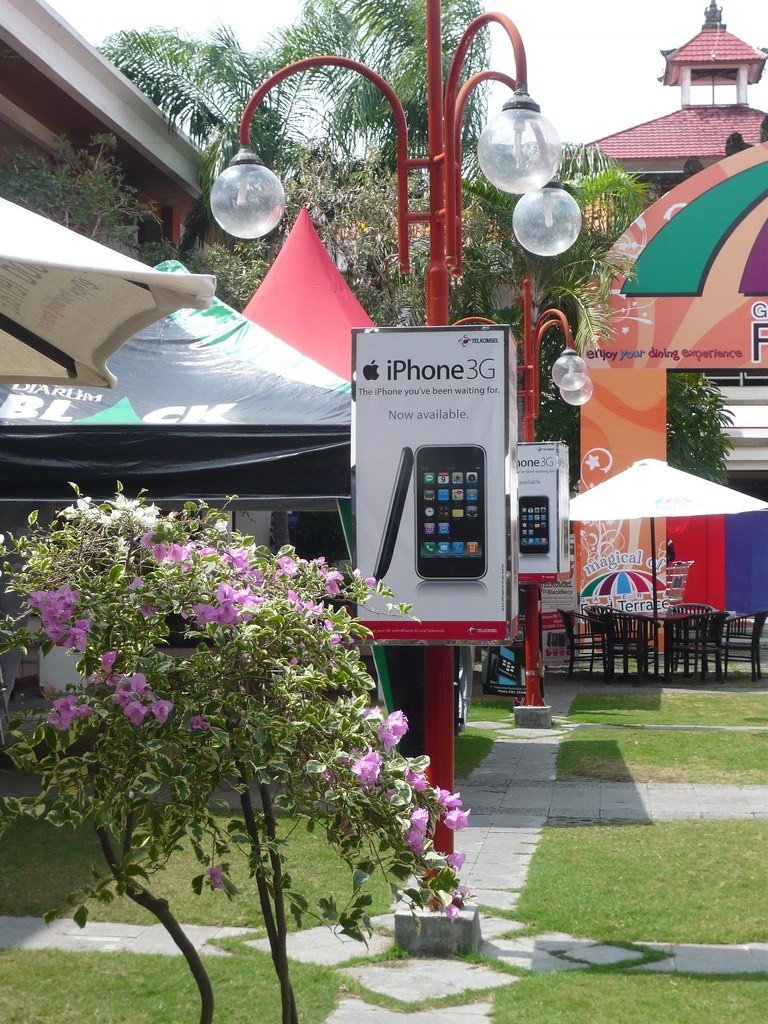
555, 603, 768, 684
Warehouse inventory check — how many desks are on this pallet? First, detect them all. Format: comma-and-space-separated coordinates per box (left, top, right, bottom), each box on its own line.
610, 611, 696, 679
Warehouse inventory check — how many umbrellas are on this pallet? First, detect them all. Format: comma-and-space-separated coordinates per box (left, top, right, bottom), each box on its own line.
0, 193, 218, 390
572, 458, 768, 675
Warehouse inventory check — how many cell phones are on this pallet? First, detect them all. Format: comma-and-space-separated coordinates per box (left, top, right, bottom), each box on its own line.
415, 443, 490, 581
372, 448, 414, 583
516, 495, 550, 557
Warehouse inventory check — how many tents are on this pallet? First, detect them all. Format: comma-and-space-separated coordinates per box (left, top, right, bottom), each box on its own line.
1, 260, 471, 756
243, 205, 377, 382
